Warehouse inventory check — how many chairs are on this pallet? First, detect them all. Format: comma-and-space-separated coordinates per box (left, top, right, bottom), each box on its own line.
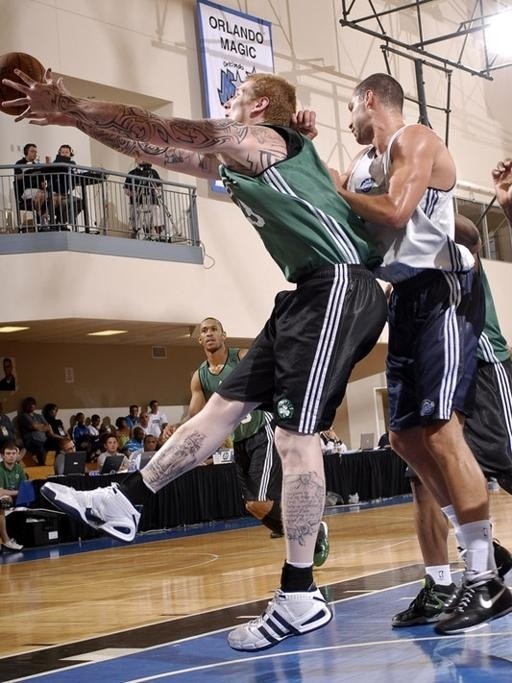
12, 179, 50, 233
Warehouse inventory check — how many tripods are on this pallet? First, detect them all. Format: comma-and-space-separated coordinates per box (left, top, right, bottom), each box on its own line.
130, 175, 184, 236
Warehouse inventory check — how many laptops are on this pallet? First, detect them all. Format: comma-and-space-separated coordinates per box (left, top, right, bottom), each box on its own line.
87, 455, 124, 474
360, 431, 374, 450
138, 451, 155, 467
47, 452, 85, 476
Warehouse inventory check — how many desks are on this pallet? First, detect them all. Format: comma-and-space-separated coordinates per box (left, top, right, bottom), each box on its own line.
5, 508, 67, 547
138, 462, 254, 533
323, 450, 411, 505
68, 170, 108, 235
31, 472, 130, 544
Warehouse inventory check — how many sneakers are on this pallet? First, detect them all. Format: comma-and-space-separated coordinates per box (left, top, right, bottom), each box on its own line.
0, 536, 24, 553
226, 587, 334, 654
391, 537, 512, 636
40, 481, 143, 543
313, 521, 331, 567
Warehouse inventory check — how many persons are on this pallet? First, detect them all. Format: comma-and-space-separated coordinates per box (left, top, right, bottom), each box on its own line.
291, 72, 512, 635
181, 317, 330, 567
391, 211, 512, 626
492, 158, 512, 229
15, 144, 171, 242
0, 359, 15, 392
0, 65, 388, 651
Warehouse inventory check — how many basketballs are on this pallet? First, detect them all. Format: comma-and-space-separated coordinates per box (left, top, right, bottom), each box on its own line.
0, 52, 46, 115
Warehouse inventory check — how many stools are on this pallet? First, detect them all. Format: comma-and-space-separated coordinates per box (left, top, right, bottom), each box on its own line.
54, 209, 79, 232
